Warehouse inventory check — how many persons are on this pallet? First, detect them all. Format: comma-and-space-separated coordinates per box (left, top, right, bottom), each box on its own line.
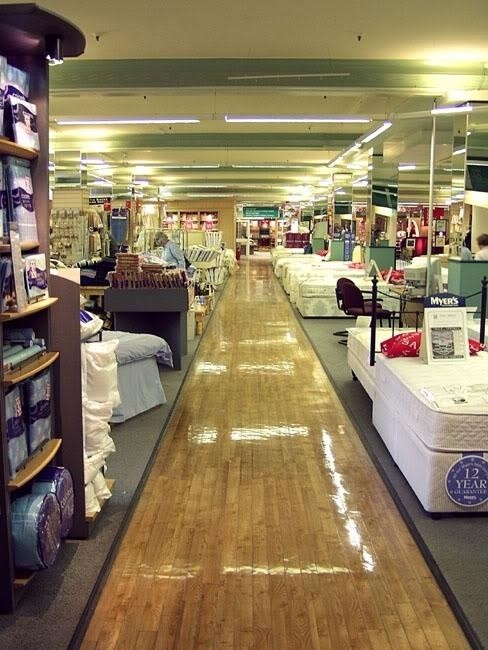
475, 233, 488, 260
155, 232, 185, 271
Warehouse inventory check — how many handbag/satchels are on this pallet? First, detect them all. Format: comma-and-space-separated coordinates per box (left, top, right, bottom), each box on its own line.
169, 244, 192, 269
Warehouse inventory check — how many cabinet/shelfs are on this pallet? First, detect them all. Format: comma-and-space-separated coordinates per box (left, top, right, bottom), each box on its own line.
0, 4, 91, 620
162, 210, 219, 231
102, 285, 195, 372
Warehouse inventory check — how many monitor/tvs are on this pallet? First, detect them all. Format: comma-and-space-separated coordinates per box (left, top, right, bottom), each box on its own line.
406, 239, 416, 249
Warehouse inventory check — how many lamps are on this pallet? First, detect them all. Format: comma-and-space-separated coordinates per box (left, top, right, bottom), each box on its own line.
53, 115, 201, 125
430, 101, 473, 116
223, 113, 372, 123
341, 140, 362, 158
326, 154, 343, 169
231, 165, 313, 170
360, 118, 393, 144
135, 164, 220, 169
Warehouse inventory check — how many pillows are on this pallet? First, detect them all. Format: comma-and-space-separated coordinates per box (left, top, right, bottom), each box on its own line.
84, 453, 113, 516
83, 400, 116, 454
81, 339, 122, 407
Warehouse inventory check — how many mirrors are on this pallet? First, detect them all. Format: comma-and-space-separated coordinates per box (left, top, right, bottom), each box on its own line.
450, 101, 488, 263
367, 142, 398, 248
332, 173, 353, 239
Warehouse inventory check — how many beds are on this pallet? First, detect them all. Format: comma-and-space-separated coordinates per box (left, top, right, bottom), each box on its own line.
343, 324, 488, 521
87, 328, 174, 425
270, 246, 457, 318
